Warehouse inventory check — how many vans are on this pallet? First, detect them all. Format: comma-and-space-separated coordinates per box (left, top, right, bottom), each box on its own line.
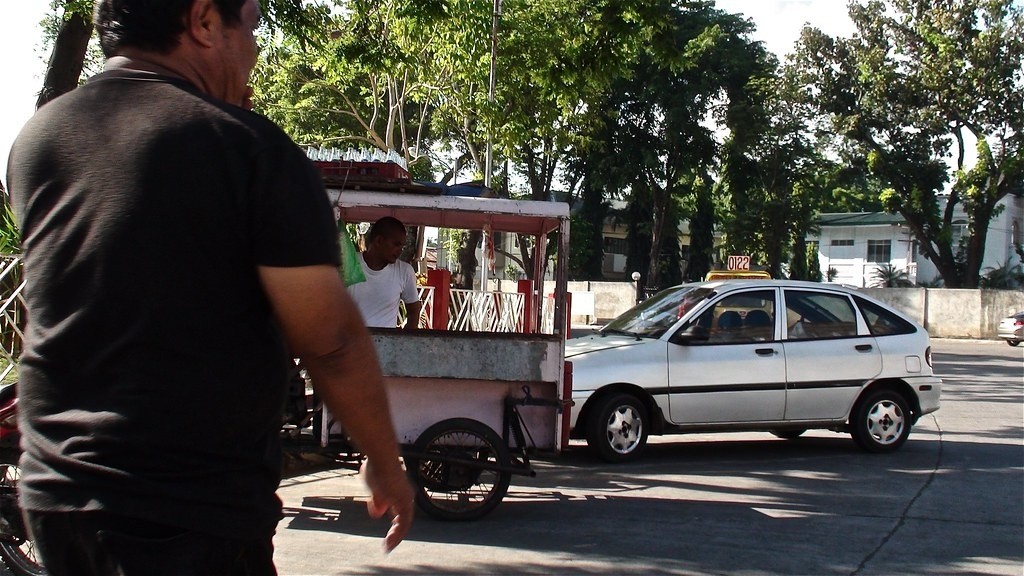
702, 269, 775, 344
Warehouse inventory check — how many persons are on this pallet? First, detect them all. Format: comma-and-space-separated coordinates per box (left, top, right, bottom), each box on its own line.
344, 216, 422, 329
6, 0, 415, 576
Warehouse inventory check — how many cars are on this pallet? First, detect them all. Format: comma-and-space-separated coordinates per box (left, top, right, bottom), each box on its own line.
556, 279, 942, 466
997, 310, 1024, 347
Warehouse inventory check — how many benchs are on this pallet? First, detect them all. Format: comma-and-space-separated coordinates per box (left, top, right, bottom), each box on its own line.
794, 321, 892, 337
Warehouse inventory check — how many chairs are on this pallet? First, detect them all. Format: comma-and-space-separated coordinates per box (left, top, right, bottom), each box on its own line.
711, 309, 773, 342
